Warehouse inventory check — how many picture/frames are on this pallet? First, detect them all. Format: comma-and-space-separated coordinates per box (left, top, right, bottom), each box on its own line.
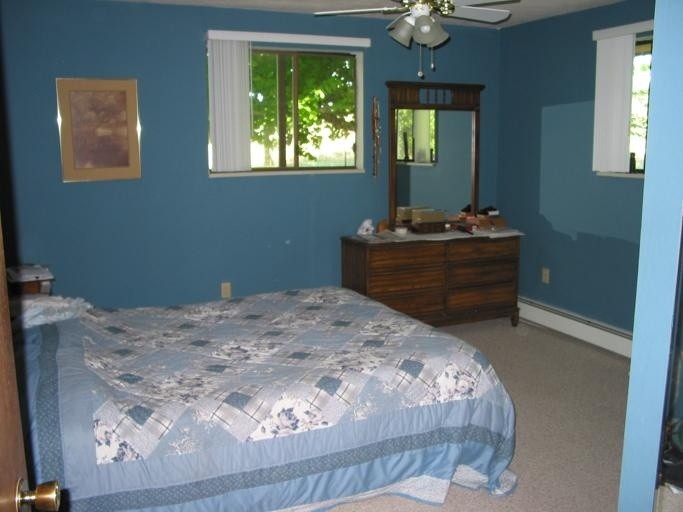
54, 75, 144, 183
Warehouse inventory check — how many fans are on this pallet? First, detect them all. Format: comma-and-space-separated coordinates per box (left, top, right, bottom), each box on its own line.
312, 0, 520, 24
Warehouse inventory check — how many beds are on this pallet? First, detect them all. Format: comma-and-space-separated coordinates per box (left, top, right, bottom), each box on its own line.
14, 286, 516, 508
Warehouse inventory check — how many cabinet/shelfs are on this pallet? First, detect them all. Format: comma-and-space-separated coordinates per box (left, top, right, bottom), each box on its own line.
343, 238, 522, 328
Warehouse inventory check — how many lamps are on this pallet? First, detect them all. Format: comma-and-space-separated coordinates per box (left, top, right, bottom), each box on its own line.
387, 0, 454, 76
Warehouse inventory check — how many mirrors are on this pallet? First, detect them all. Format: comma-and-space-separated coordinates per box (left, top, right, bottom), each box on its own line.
386, 83, 485, 220
